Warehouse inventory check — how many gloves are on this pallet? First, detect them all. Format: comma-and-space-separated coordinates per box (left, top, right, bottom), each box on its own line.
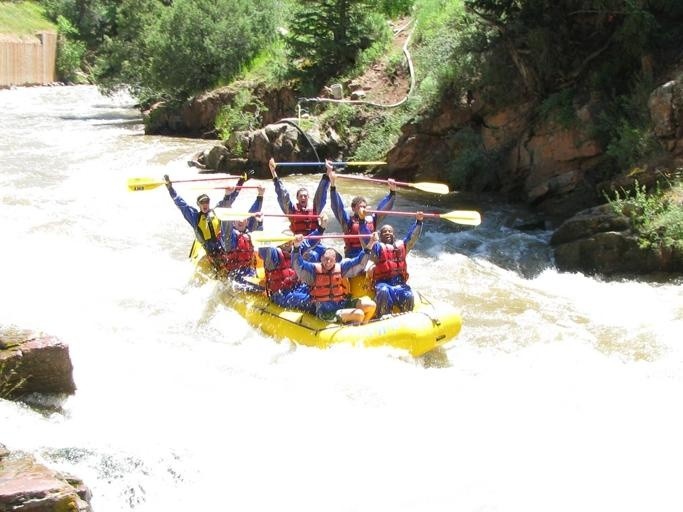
162, 174, 175, 193
238, 168, 255, 185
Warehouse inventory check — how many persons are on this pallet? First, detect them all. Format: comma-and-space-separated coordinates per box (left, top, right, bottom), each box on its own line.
220, 185, 264, 292
163, 170, 256, 258
268, 157, 334, 235
290, 232, 381, 326
327, 171, 397, 258
356, 206, 424, 319
252, 211, 329, 311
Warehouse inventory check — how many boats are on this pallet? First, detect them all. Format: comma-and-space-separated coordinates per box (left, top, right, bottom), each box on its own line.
192, 241, 463, 358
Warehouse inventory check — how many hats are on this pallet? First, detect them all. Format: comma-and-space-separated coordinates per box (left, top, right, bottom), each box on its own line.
196, 193, 210, 202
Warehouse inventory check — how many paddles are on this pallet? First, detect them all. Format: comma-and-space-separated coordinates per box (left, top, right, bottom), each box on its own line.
273, 162, 388, 168
337, 174, 449, 194
214, 207, 329, 221
363, 209, 482, 226
255, 234, 371, 243
127, 172, 248, 191
